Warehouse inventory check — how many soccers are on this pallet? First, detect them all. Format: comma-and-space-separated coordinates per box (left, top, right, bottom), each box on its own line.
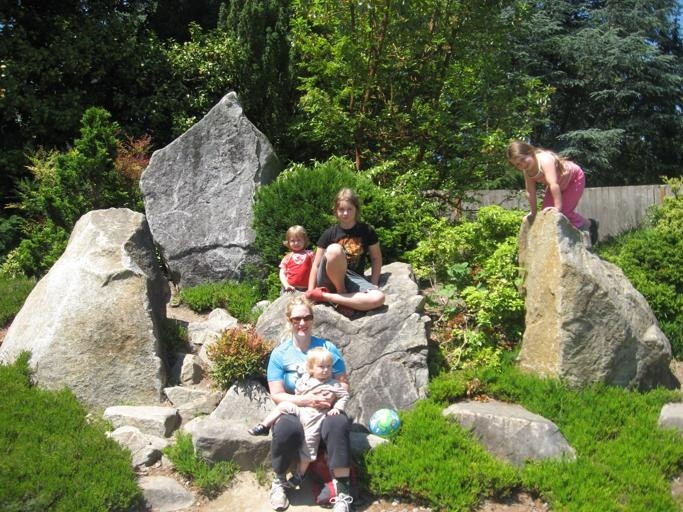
369, 408, 401, 438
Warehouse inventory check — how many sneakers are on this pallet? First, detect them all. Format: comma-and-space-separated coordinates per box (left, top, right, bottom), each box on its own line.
288, 471, 305, 487
248, 422, 270, 436
588, 218, 599, 244
328, 493, 353, 512
269, 478, 290, 511
337, 304, 353, 317
305, 286, 329, 301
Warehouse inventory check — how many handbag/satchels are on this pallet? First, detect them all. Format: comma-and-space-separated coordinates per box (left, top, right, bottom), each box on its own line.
311, 452, 358, 505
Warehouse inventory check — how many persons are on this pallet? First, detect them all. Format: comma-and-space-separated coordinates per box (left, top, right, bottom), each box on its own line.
505, 142, 599, 246
250, 347, 350, 488
267, 296, 354, 512
279, 225, 316, 295
305, 187, 385, 317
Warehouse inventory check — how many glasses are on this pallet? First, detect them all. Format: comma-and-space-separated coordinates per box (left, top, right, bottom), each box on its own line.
290, 314, 313, 322
